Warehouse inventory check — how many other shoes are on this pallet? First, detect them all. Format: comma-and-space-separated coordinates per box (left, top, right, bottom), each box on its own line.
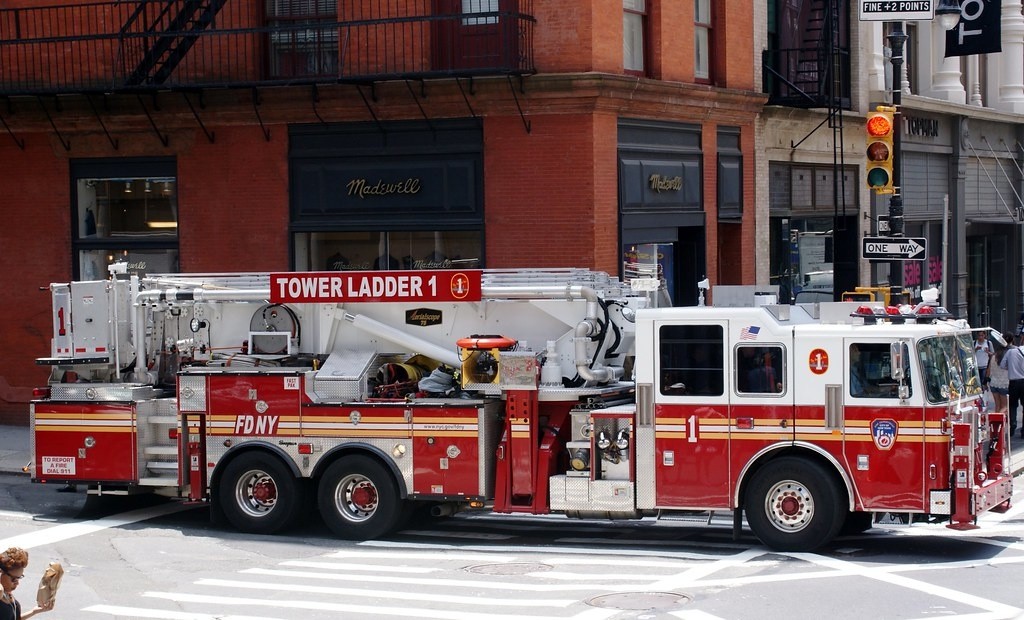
1010, 424, 1017, 437
1021, 429, 1024, 439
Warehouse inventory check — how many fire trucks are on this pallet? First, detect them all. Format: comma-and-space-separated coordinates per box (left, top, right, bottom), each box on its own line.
24, 237, 1013, 553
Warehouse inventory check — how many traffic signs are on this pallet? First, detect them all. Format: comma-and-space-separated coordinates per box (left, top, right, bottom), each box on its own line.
862, 238, 928, 260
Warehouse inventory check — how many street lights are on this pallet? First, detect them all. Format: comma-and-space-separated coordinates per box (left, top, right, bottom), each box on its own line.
890, 0, 964, 306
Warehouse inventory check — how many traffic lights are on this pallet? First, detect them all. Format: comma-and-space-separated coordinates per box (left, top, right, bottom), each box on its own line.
864, 108, 897, 194
842, 291, 875, 302
888, 287, 911, 306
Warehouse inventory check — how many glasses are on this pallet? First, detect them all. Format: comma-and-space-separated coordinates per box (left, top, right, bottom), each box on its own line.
6, 572, 24, 582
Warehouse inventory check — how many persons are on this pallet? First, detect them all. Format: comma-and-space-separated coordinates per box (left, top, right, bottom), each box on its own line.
965, 314, 1024, 447
0, 547, 56, 620
850, 344, 897, 398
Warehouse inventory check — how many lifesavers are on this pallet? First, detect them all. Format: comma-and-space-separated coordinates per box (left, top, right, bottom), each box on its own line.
455, 332, 517, 352
